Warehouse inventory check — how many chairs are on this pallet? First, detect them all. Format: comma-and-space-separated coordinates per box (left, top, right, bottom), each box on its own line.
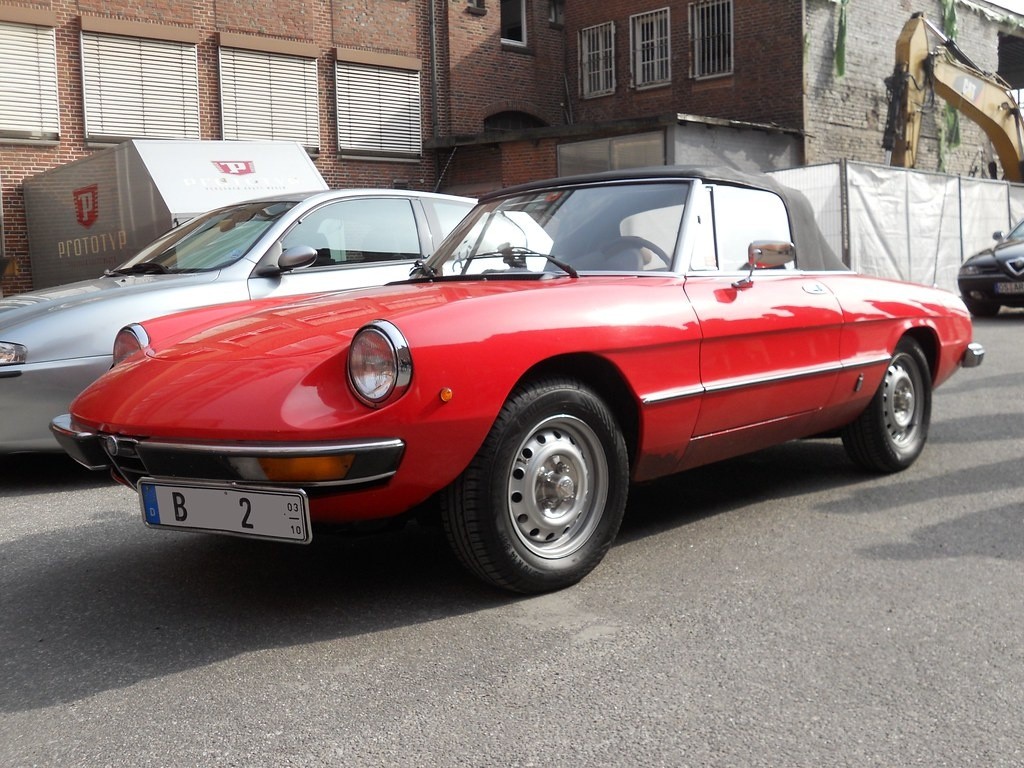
561, 245, 643, 271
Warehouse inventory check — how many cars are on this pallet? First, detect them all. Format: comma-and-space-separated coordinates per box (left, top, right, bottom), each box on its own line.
0, 187, 554, 456
957, 218, 1024, 316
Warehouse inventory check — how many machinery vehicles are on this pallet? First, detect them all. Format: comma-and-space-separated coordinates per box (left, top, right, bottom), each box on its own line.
879, 10, 1024, 187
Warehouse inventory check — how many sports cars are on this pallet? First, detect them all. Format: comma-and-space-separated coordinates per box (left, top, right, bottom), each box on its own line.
47, 163, 990, 599
